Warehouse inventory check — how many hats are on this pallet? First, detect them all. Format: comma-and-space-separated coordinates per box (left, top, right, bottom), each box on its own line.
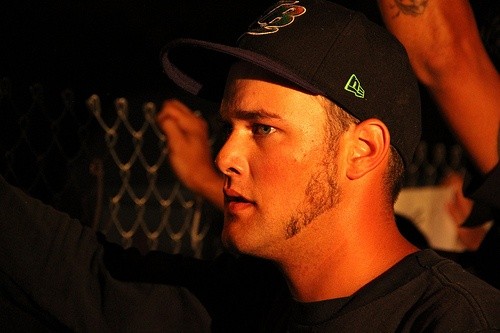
161, 0, 421, 171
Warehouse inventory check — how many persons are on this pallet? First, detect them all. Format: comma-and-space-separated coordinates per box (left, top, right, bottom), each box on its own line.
157, 0, 500, 291
0, 0, 500, 333
